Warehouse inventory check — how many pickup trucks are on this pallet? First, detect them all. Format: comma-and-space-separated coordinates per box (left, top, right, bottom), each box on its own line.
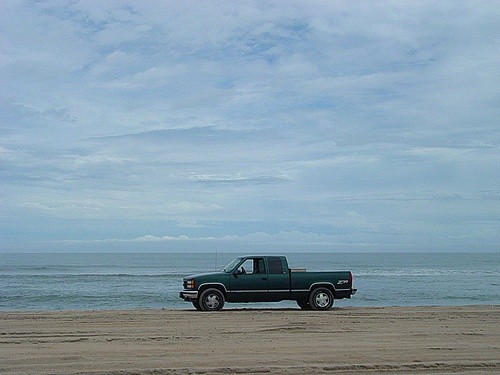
179, 255, 357, 311
